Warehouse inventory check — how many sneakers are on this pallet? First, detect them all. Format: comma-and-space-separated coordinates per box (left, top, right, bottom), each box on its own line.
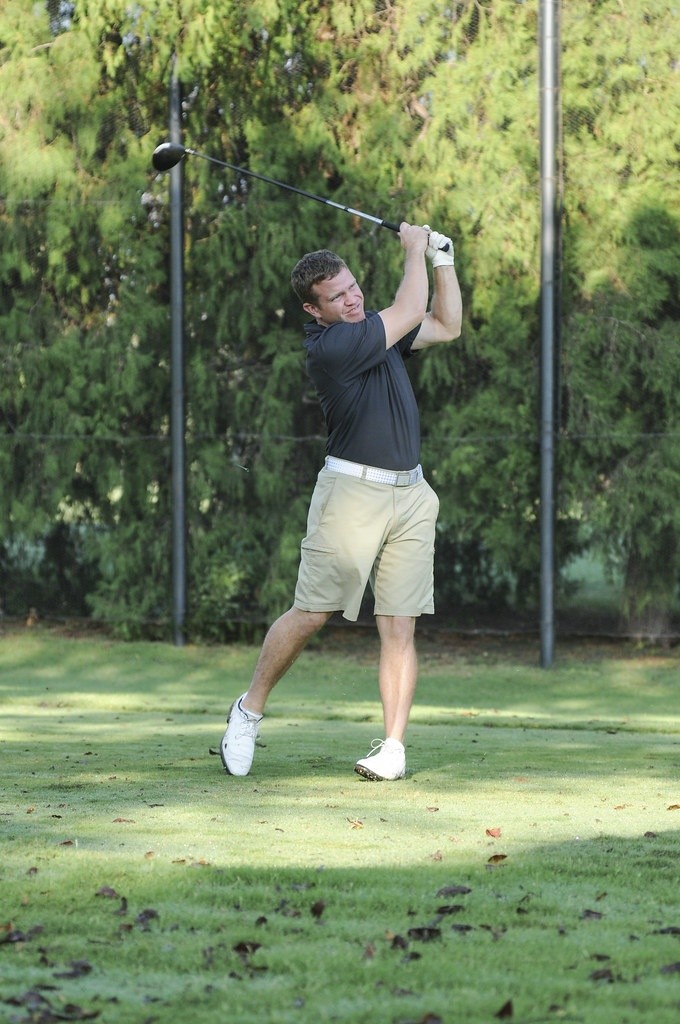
353, 739, 406, 781
220, 693, 264, 776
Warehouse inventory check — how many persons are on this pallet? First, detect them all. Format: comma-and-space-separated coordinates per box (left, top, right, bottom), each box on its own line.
219, 221, 463, 782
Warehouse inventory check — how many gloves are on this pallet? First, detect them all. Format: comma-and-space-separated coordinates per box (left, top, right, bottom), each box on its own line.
423, 224, 454, 268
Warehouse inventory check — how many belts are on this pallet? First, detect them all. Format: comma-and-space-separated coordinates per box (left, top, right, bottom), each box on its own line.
325, 456, 423, 487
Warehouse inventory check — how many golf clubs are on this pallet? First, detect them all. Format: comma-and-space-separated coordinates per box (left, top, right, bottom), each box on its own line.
152, 142, 450, 252
